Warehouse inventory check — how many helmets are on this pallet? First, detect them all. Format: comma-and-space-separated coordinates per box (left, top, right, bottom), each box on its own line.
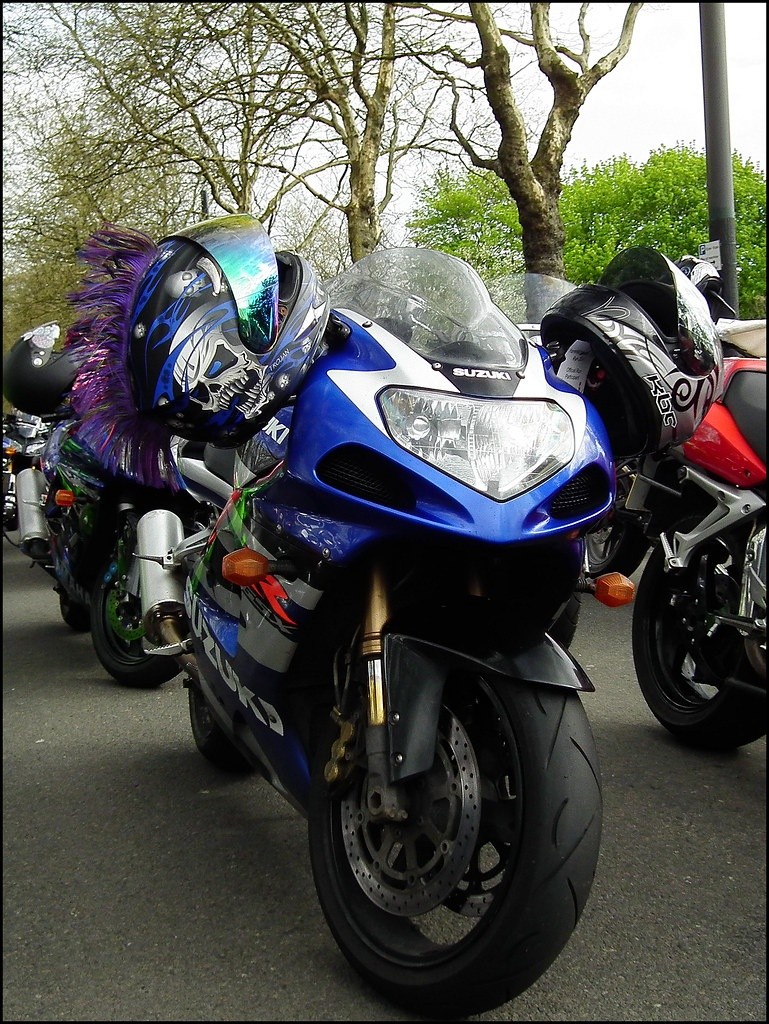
63, 213, 329, 488
3, 318, 91, 416
540, 246, 723, 458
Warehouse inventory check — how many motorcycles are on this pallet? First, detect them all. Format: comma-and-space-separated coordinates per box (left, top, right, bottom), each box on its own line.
0, 242, 768, 1021
